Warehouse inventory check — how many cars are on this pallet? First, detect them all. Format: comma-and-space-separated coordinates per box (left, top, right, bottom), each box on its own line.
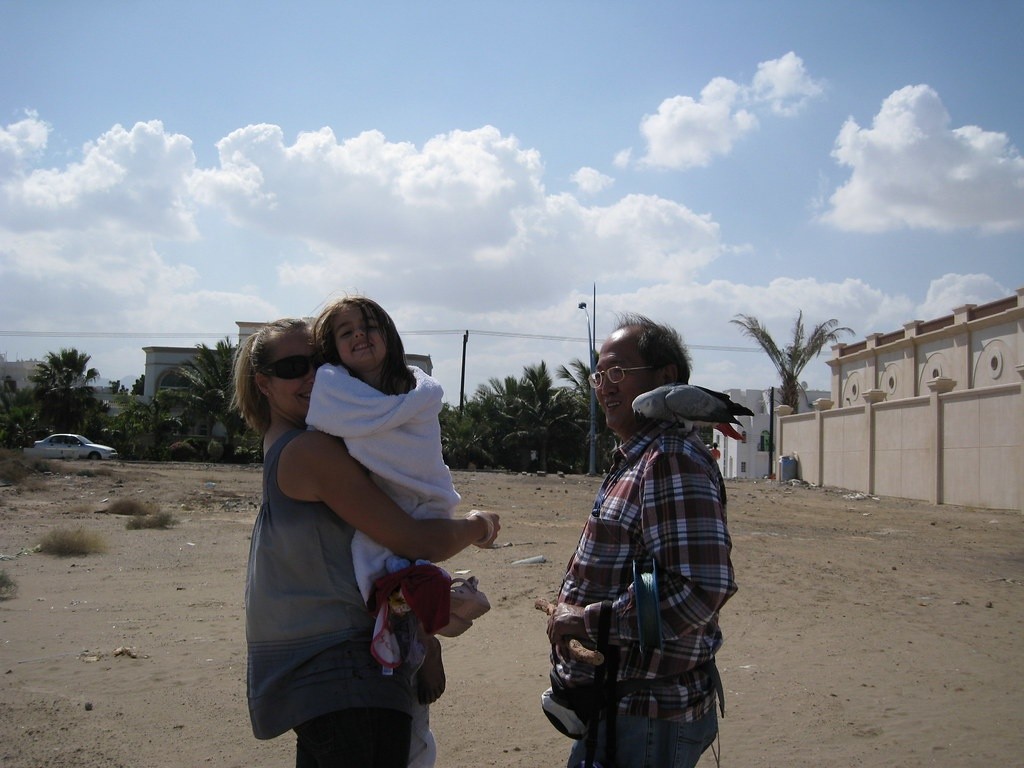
34, 434, 118, 460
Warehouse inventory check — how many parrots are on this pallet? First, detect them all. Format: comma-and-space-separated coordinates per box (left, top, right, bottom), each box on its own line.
632, 381, 756, 444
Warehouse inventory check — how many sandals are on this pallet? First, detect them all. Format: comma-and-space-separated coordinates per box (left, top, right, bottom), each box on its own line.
419, 576, 491, 637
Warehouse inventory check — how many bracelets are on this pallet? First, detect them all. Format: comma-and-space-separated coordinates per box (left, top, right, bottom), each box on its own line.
468, 511, 493, 547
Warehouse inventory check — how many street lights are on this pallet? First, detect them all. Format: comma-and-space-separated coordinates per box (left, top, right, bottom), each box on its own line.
579, 282, 597, 474
460, 330, 469, 414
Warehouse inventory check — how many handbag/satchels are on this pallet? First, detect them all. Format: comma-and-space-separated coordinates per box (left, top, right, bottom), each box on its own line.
541, 666, 671, 741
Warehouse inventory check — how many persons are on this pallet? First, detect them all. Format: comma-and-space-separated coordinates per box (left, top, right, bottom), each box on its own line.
709, 443, 720, 462
231, 294, 501, 768
545, 312, 738, 768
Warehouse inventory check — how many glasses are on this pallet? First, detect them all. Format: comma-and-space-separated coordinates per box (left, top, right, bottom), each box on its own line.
588, 363, 669, 389
256, 351, 327, 379
